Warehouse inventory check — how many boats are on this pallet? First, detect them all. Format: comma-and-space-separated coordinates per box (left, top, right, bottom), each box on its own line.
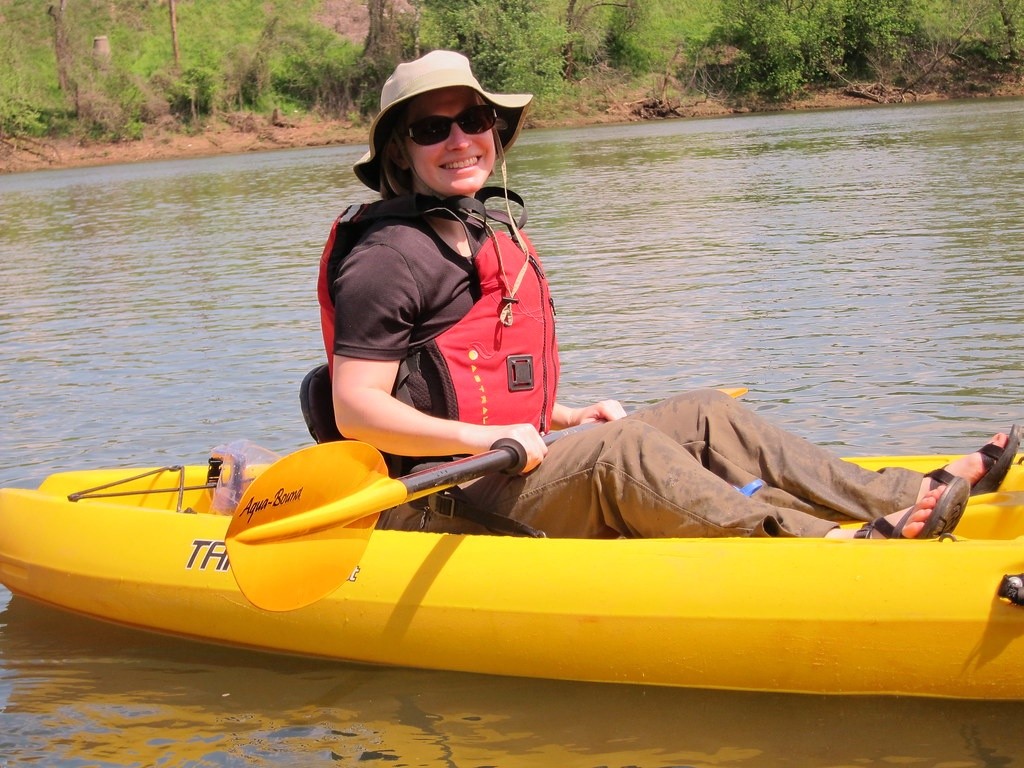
0, 447, 1024, 703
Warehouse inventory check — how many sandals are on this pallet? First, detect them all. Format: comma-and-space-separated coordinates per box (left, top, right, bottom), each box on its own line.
920, 424, 1022, 496
852, 477, 969, 539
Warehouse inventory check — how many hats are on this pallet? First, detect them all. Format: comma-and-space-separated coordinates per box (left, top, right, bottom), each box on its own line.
353, 50, 534, 192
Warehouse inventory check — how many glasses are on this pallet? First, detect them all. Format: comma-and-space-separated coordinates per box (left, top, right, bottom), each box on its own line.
397, 104, 498, 146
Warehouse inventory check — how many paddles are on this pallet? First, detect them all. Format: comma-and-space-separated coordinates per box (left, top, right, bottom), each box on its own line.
225, 385, 752, 612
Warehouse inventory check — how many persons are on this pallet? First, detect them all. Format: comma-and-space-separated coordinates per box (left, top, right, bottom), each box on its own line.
316, 49, 1021, 538
